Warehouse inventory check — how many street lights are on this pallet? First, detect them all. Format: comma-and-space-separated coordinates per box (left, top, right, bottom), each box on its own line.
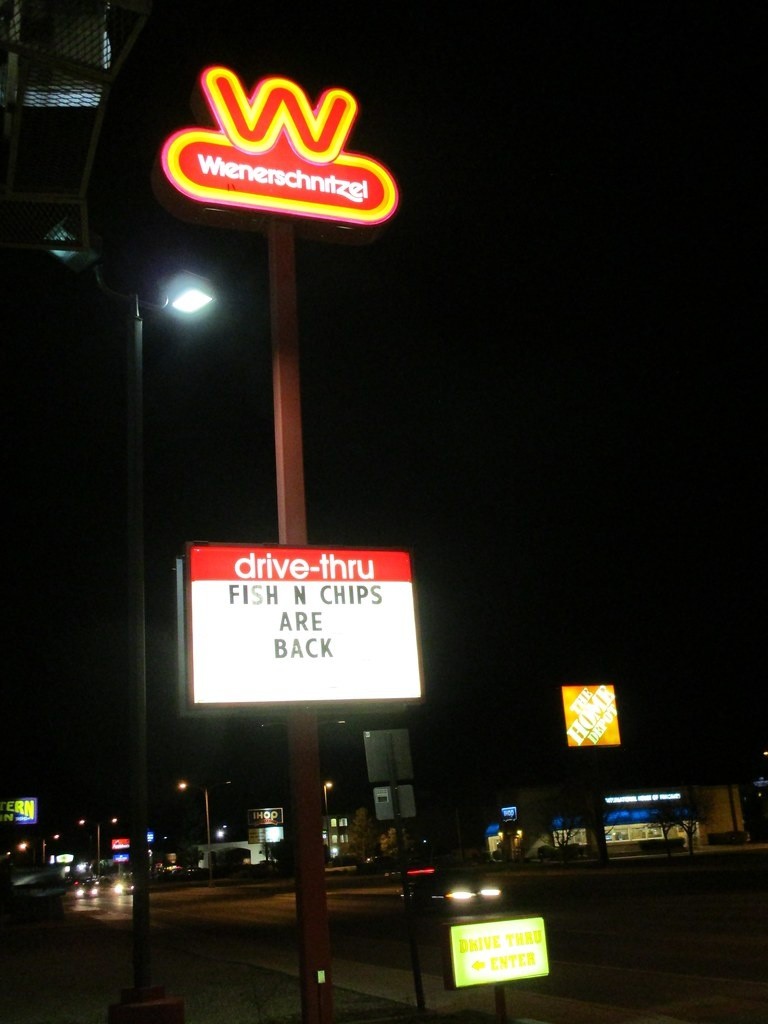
323, 780, 334, 866
18, 834, 60, 864
78, 817, 117, 876
39, 219, 216, 1024
177, 780, 232, 889
